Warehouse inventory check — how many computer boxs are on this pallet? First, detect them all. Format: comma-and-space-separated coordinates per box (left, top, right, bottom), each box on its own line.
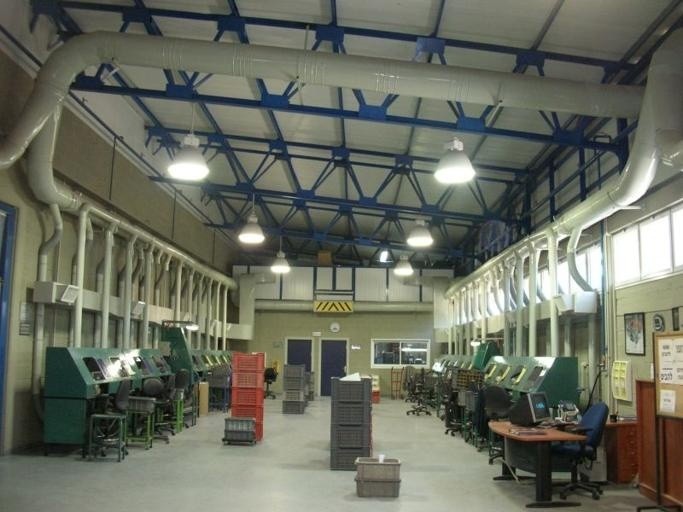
579, 447, 607, 482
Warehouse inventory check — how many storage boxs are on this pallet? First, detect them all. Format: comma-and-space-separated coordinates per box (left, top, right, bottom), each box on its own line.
352, 454, 400, 501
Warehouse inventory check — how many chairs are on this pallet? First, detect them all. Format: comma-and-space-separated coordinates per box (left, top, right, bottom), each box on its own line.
560, 397, 608, 502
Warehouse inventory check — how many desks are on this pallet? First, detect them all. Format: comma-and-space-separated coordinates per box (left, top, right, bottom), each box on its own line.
487, 418, 587, 509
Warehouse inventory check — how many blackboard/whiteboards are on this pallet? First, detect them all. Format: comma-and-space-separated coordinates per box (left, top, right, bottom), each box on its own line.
653, 333, 683, 420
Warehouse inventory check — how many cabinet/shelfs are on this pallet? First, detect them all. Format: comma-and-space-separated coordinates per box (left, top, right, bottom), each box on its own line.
607, 425, 637, 485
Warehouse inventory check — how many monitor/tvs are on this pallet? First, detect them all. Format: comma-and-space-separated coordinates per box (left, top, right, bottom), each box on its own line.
441, 360, 473, 370
511, 392, 553, 427
192, 355, 231, 365
485, 364, 543, 382
83, 355, 169, 381
